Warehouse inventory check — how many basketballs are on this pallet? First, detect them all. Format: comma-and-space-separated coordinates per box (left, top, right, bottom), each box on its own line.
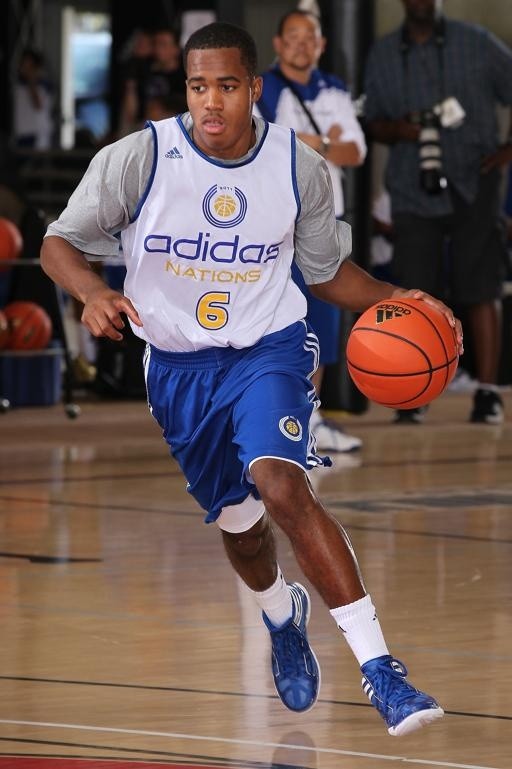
5, 303, 52, 349
346, 298, 459, 410
0, 218, 22, 258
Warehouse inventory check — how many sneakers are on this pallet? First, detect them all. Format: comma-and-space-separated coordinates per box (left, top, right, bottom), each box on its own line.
309, 422, 362, 452
360, 655, 444, 736
393, 407, 426, 422
469, 389, 502, 424
262, 582, 321, 714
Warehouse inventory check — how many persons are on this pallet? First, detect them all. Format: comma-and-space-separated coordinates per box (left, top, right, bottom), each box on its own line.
1, 0, 512, 451
41, 23, 446, 739
